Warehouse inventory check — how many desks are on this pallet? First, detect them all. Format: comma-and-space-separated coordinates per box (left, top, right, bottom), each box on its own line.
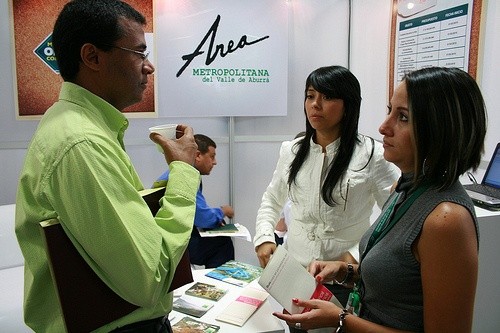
166, 277, 290, 333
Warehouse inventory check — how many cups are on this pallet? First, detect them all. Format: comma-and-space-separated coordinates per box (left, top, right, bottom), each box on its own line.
149, 124, 178, 152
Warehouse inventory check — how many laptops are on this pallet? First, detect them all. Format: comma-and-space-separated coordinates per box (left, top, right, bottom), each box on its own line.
463, 143, 500, 209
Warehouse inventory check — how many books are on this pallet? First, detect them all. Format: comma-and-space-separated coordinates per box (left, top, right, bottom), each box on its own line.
215, 287, 270, 326
38, 187, 194, 333
172, 296, 214, 318
258, 244, 344, 315
205, 260, 264, 287
171, 316, 220, 333
199, 223, 239, 233
185, 282, 230, 302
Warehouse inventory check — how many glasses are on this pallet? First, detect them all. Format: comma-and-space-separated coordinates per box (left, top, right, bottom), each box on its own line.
115, 41, 150, 61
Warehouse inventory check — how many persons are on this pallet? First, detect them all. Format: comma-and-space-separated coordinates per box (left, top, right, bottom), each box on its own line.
274, 198, 293, 231
15, 0, 201, 333
272, 66, 488, 333
254, 65, 403, 308
150, 134, 235, 268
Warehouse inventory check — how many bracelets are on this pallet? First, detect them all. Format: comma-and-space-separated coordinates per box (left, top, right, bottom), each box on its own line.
339, 309, 348, 330
333, 263, 354, 286
